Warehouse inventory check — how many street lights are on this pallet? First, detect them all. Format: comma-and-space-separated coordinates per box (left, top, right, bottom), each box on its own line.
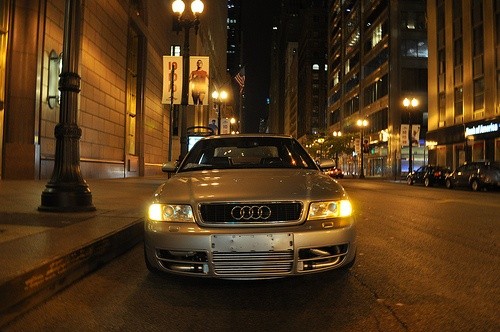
357, 120, 368, 179
318, 138, 324, 159
212, 90, 227, 134
402, 97, 419, 173
333, 131, 342, 168
171, 0, 205, 172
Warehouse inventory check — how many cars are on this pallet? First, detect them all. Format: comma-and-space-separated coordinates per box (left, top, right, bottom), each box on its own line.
445, 164, 500, 192
142, 134, 359, 282
406, 166, 453, 188
323, 169, 344, 179
